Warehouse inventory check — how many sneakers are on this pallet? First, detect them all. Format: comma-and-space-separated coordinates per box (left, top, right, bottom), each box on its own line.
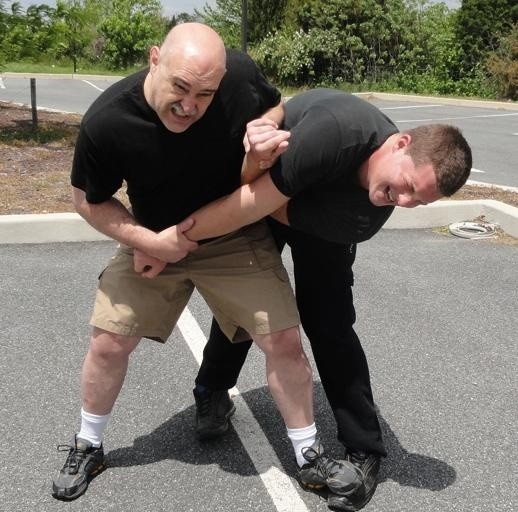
328, 446, 381, 512
193, 387, 236, 439
294, 437, 365, 496
53, 433, 106, 501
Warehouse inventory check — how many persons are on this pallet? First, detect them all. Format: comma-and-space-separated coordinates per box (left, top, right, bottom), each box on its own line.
132, 87, 473, 511
52, 17, 364, 501
